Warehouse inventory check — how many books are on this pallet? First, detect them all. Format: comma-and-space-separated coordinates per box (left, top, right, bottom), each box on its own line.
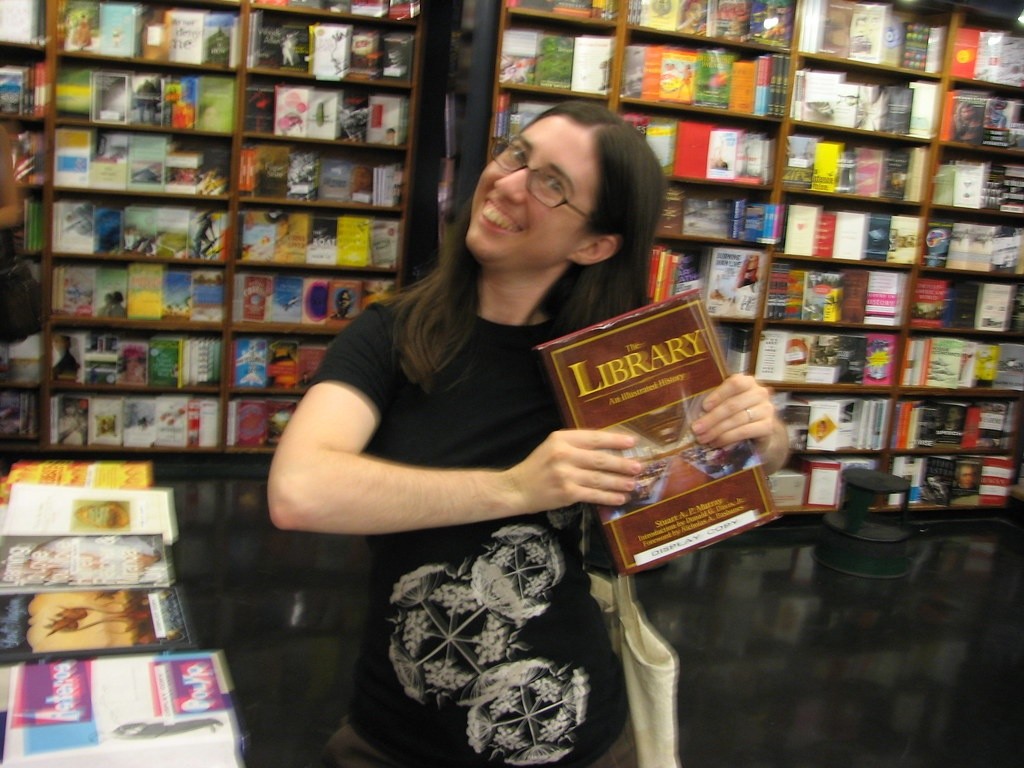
0, 391, 299, 450
496, 1, 1024, 151
6, 129, 405, 209
0, 0, 421, 78
648, 244, 1024, 334
534, 289, 781, 576
708, 323, 1024, 389
803, 456, 1024, 508
0, 334, 328, 390
655, 186, 1024, 275
772, 389, 1022, 453
0, 60, 410, 146
51, 263, 395, 325
0, 461, 244, 768
645, 120, 1024, 214
20, 196, 399, 270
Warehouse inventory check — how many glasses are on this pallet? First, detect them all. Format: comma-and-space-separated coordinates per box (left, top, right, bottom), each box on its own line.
488, 136, 611, 233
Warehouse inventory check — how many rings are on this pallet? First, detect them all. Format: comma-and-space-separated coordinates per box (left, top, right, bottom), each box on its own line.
745, 407, 753, 423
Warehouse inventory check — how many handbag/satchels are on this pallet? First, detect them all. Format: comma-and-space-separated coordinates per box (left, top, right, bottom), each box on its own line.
582, 571, 679, 768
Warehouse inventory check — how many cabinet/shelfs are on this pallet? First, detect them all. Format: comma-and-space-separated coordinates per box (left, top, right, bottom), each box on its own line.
0, 0, 1024, 513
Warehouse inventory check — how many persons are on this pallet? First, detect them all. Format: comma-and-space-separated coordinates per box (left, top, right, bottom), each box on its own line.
955, 464, 976, 491
380, 127, 396, 145
0, 125, 21, 273
266, 102, 789, 768
